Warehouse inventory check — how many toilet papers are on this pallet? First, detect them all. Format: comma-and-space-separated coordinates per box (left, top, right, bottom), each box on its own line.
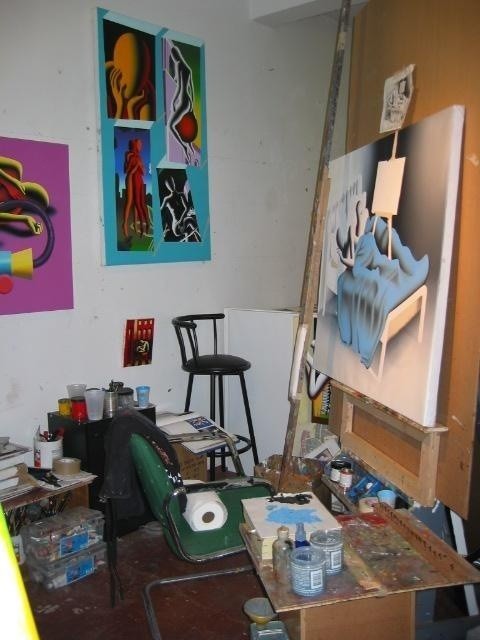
181, 478, 227, 530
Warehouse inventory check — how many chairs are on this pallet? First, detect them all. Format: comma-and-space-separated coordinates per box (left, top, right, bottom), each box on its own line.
172, 312, 262, 482
98, 409, 181, 613
131, 434, 275, 638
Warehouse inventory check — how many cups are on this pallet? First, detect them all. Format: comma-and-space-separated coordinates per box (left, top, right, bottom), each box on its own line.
84, 390, 106, 421
67, 384, 86, 399
71, 396, 87, 423
58, 399, 71, 416
136, 385, 150, 409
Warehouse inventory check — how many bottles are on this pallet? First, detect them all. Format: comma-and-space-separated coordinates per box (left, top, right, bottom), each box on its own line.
272, 526, 295, 584
339, 463, 352, 492
309, 527, 344, 575
294, 523, 311, 563
290, 545, 328, 597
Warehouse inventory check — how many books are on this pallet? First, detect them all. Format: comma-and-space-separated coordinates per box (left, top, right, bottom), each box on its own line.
0, 437, 40, 507
155, 407, 240, 452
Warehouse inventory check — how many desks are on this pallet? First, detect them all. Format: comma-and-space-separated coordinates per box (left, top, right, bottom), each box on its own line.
237, 507, 480, 640
0, 404, 157, 535
